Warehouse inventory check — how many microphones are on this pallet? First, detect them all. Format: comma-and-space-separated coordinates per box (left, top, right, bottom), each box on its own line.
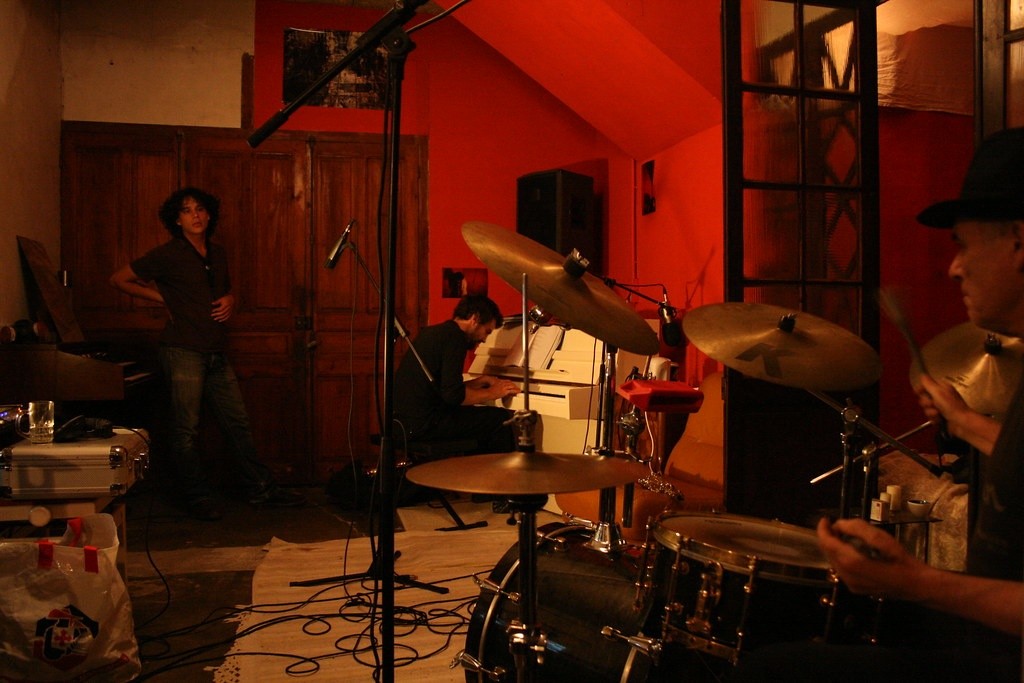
660, 288, 682, 347
325, 221, 354, 269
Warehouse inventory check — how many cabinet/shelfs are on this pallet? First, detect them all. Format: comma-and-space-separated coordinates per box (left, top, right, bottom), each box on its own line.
54, 118, 425, 485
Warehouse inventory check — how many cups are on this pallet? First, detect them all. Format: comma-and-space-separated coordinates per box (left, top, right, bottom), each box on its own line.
881, 484, 901, 510
16, 401, 54, 442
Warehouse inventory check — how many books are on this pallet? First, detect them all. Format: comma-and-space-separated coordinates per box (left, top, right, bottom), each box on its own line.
502, 322, 563, 371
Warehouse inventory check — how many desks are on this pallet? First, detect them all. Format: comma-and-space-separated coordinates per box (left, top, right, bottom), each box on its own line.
0, 496, 140, 590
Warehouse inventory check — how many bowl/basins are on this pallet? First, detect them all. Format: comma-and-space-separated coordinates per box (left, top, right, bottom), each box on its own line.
907, 500, 931, 517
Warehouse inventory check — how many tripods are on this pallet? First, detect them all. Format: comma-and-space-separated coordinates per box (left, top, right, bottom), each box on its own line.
289, 240, 449, 594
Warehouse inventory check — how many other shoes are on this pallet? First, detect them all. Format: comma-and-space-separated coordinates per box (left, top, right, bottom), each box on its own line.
251, 486, 304, 509
472, 492, 518, 514
190, 493, 222, 523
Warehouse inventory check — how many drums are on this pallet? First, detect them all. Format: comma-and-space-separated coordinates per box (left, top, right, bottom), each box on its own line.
464, 521, 756, 683
646, 512, 840, 666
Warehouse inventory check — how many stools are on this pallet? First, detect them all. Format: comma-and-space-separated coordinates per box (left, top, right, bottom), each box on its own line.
364, 434, 490, 533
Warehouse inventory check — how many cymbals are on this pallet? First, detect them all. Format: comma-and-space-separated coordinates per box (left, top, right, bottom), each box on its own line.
682, 301, 884, 391
458, 218, 662, 356
405, 450, 654, 496
908, 321, 1023, 415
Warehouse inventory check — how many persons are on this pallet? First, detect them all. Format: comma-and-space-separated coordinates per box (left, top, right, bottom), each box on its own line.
391, 291, 521, 503
817, 125, 1024, 683
108, 184, 307, 520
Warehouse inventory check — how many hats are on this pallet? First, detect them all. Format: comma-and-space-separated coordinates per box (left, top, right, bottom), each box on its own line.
914, 126, 1024, 230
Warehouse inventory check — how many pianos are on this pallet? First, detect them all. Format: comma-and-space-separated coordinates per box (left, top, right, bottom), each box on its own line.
0, 235, 166, 411
463, 316, 661, 455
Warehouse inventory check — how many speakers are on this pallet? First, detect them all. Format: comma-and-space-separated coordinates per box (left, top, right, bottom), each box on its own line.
516, 167, 600, 278
